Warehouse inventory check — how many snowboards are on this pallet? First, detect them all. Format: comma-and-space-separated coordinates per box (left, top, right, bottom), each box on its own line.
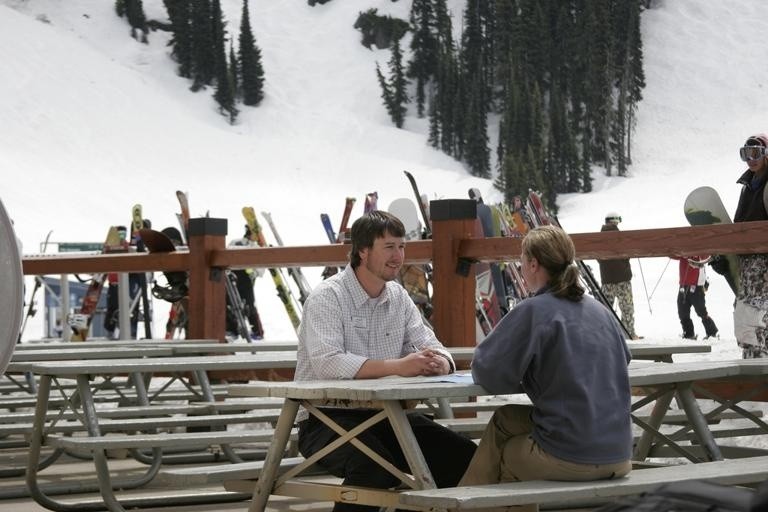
685, 185, 741, 294
384, 198, 433, 324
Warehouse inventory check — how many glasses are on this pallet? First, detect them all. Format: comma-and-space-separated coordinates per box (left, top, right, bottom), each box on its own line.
740, 145, 763, 161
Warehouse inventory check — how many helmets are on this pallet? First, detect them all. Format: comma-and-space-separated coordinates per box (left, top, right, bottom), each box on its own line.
605, 212, 623, 224
739, 134, 768, 162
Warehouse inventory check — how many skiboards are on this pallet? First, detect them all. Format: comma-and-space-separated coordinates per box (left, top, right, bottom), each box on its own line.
468, 188, 631, 342
322, 198, 355, 279
69, 191, 190, 342
19, 228, 53, 342
404, 171, 491, 335
226, 207, 309, 340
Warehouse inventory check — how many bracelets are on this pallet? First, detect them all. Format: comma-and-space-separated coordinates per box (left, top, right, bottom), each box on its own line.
441, 355, 454, 374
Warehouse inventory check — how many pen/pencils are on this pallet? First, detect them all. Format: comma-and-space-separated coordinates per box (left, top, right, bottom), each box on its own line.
412, 344, 423, 352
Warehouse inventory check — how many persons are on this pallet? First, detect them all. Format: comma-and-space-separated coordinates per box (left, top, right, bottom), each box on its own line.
511, 195, 522, 210
709, 133, 768, 357
596, 213, 640, 339
229, 225, 264, 287
294, 210, 480, 512
458, 226, 633, 512
669, 254, 720, 340
